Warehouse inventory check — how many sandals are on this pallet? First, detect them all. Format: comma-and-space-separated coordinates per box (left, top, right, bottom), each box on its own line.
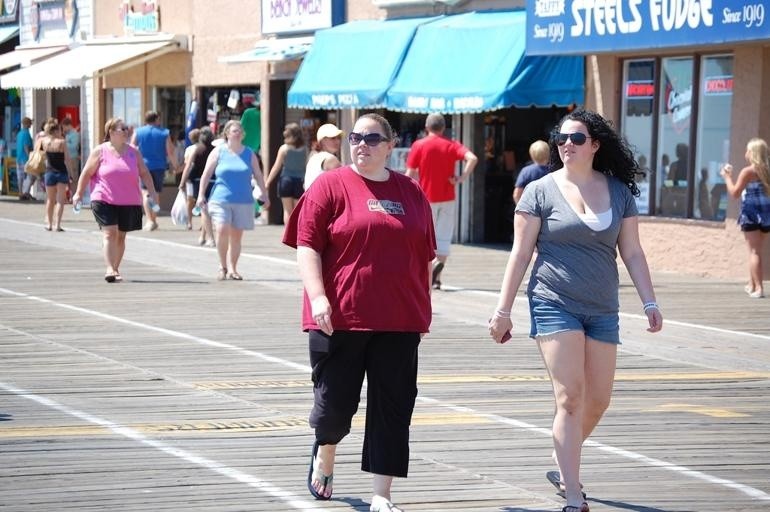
218, 268, 242, 281
432, 260, 443, 289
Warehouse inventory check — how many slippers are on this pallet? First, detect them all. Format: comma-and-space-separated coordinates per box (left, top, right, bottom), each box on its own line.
546, 471, 589, 512
307, 439, 333, 499
369, 503, 403, 512
105, 271, 121, 282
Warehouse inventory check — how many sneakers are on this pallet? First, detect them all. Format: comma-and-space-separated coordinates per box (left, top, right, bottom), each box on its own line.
195, 237, 216, 248
142, 221, 157, 232
745, 284, 763, 299
19, 193, 37, 201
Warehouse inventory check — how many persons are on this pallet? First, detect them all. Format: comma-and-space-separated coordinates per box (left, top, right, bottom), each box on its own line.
488, 109, 662, 511
404, 113, 478, 289
131, 113, 176, 231
264, 122, 309, 226
282, 114, 438, 512
513, 140, 554, 253
721, 138, 769, 299
635, 144, 715, 222
169, 126, 216, 247
196, 120, 271, 281
304, 124, 344, 191
72, 118, 159, 282
16, 118, 80, 231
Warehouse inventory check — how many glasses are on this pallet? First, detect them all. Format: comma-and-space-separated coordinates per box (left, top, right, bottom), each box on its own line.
554, 133, 593, 146
348, 132, 392, 147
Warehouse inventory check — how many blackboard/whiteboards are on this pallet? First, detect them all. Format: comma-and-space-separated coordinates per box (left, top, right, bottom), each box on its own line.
3, 157, 19, 196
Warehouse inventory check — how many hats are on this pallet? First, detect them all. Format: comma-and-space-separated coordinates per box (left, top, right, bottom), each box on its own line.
317, 124, 343, 143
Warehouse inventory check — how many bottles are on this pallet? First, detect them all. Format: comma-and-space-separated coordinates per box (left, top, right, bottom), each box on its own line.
145, 193, 161, 213
191, 199, 208, 217
255, 199, 264, 219
72, 202, 83, 216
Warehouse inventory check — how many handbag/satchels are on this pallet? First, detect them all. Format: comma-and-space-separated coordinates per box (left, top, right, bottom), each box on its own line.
26, 150, 46, 176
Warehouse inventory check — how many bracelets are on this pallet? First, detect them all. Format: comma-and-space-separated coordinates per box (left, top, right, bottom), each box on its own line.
495, 308, 511, 319
643, 303, 659, 313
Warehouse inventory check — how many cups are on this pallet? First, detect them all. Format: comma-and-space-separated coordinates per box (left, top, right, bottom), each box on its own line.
720, 163, 734, 178
